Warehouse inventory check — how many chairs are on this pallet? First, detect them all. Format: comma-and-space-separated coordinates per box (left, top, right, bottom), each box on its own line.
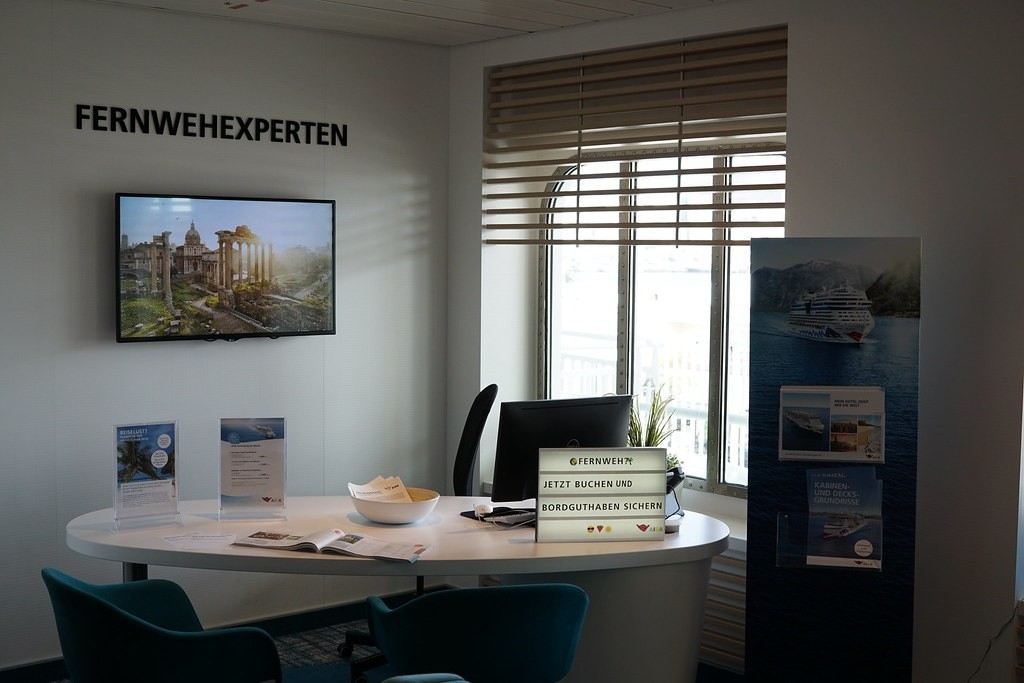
366, 584, 590, 683
339, 383, 499, 683
41, 568, 282, 683
383, 673, 470, 683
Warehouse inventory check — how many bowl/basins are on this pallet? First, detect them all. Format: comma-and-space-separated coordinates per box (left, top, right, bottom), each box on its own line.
351, 486, 440, 524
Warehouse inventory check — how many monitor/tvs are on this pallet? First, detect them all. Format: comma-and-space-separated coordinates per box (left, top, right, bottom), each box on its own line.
115, 192, 336, 342
491, 394, 633, 502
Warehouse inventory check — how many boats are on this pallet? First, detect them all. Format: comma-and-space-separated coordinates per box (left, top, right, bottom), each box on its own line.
822, 511, 871, 541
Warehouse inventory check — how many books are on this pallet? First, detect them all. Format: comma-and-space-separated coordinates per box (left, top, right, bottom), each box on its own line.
233, 528, 431, 565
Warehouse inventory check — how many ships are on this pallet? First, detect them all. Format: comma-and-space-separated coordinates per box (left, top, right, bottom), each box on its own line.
786, 279, 875, 344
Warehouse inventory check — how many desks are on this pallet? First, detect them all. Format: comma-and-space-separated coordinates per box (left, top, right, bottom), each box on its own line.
65, 495, 729, 683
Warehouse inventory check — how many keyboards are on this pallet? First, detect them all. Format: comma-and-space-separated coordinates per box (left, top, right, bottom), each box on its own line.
483, 511, 536, 525
